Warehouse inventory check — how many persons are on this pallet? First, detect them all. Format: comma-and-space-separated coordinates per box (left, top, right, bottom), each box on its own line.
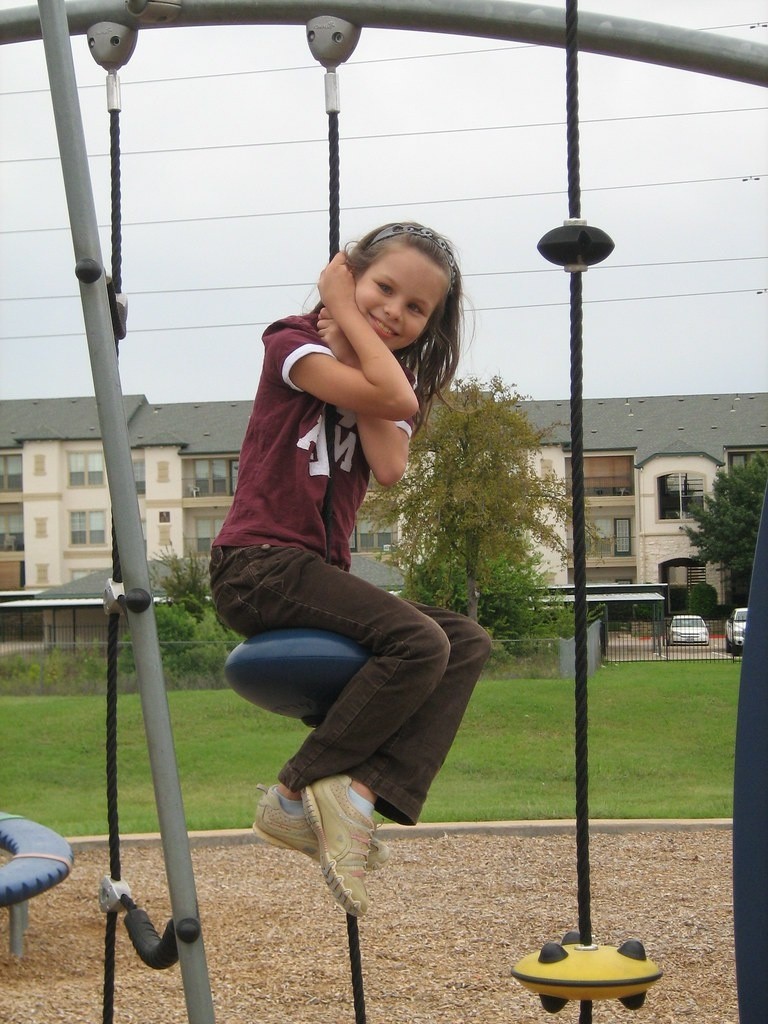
207, 221, 492, 918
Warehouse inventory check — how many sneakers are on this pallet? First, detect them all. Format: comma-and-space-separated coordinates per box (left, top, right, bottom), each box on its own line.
300, 774, 384, 917
253, 784, 390, 872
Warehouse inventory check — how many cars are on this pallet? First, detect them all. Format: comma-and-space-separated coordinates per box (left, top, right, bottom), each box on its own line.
667, 615, 709, 646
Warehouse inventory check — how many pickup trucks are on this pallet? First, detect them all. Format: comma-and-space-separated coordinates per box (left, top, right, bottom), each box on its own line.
725, 608, 748, 656
